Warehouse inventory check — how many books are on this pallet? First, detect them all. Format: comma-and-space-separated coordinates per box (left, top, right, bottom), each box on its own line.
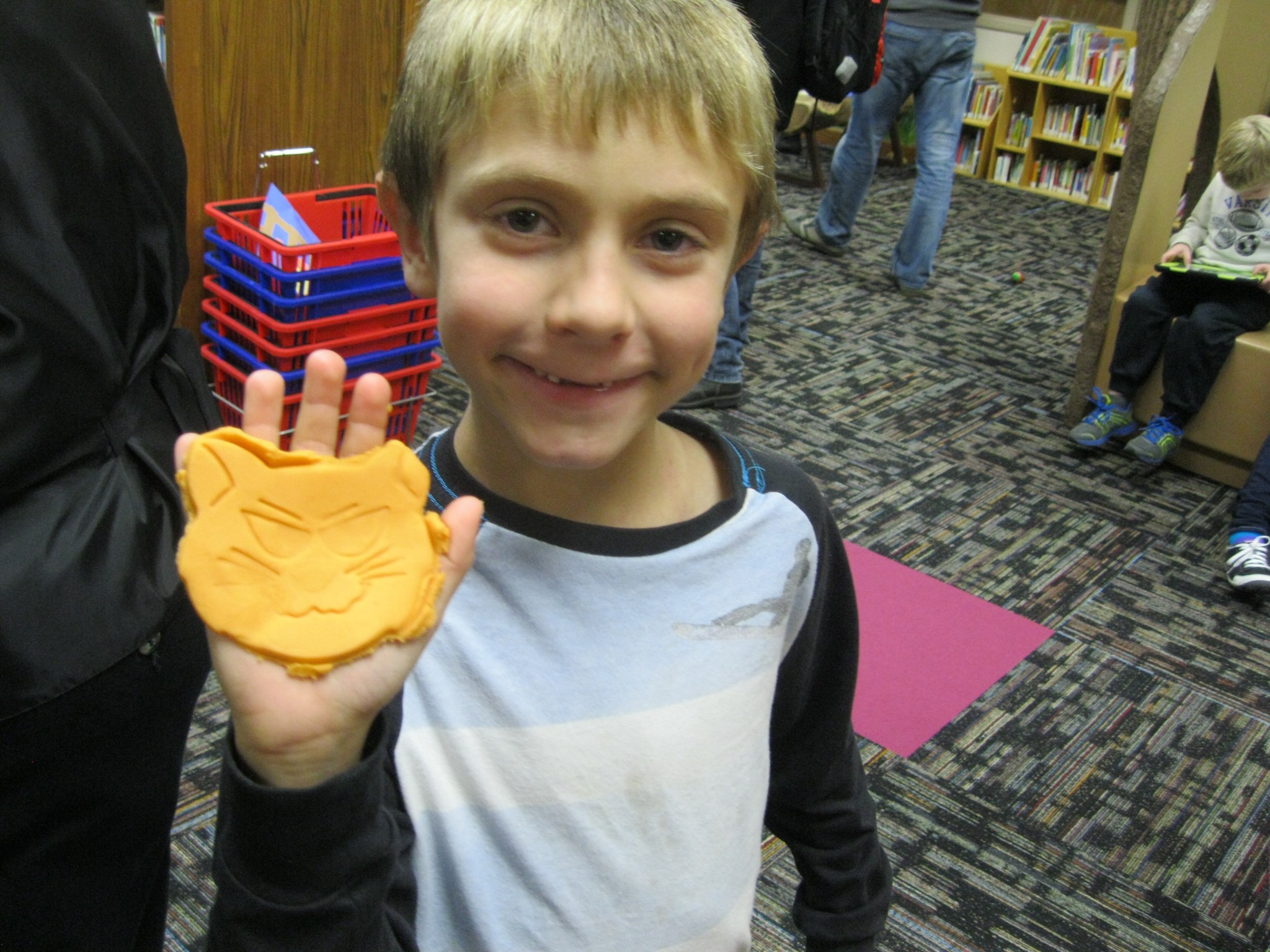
911, 12, 1201, 229
145, 10, 171, 75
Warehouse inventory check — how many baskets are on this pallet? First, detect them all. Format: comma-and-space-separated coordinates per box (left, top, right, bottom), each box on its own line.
197, 183, 441, 453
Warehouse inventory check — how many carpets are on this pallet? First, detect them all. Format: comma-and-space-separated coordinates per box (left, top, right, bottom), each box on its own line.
843, 539, 1059, 761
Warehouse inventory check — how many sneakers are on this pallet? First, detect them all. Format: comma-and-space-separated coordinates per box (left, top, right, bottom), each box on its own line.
1123, 417, 1183, 466
782, 208, 845, 257
882, 261, 922, 297
1223, 541, 1270, 591
1067, 394, 1139, 447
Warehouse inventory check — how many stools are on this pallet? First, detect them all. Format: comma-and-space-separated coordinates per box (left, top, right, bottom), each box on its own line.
1087, 265, 1269, 489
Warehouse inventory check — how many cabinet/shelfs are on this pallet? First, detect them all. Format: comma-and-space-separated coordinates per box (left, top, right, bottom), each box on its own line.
875, 14, 1198, 228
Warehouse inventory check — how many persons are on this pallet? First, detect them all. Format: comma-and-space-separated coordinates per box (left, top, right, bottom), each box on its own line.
0, 0, 231, 952
1223, 430, 1270, 589
1065, 106, 1270, 467
776, 0, 986, 301
161, 0, 899, 951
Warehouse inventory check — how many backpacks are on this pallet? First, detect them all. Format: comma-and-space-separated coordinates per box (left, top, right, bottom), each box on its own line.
799, 0, 889, 105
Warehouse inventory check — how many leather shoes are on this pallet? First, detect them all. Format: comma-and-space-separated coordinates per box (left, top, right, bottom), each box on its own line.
668, 377, 742, 410
